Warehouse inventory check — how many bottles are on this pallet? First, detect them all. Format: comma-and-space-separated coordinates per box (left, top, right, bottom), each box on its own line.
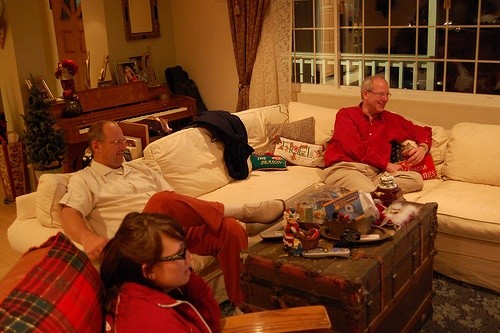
372, 173, 402, 208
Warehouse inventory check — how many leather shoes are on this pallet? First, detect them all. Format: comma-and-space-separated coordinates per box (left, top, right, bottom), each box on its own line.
239, 199, 286, 224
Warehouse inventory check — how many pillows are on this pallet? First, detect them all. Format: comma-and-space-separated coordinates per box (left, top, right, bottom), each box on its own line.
251, 153, 287, 172
267, 116, 314, 147
273, 137, 324, 167
397, 141, 438, 180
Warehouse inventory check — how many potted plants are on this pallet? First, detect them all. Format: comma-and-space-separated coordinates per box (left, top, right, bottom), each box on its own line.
20, 90, 67, 182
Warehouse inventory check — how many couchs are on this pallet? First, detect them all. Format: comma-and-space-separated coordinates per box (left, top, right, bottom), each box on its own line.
7, 100, 500, 304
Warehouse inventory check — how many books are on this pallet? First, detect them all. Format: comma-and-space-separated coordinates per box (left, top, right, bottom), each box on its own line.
122, 135, 143, 162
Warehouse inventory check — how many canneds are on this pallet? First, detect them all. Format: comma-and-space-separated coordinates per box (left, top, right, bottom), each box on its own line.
299, 200, 314, 222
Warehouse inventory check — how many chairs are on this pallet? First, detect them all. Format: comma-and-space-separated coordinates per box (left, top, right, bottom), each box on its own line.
0, 233, 332, 332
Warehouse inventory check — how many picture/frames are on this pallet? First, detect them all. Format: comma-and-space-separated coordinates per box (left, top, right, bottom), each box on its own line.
122, 0, 160, 41
128, 55, 157, 82
114, 60, 140, 84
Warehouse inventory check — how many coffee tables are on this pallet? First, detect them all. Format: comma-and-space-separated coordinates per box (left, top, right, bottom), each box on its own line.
241, 187, 440, 333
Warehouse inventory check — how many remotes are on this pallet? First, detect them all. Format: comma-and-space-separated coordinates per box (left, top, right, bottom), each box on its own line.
302, 248, 350, 258
260, 231, 285, 241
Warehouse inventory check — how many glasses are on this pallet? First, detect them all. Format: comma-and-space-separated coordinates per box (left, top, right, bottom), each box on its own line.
366, 89, 391, 98
95, 138, 127, 146
156, 243, 187, 261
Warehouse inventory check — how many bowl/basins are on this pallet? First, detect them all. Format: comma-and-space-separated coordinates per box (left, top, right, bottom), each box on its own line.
324, 214, 373, 234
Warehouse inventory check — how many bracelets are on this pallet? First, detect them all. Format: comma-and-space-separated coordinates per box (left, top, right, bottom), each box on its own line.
418, 145, 428, 152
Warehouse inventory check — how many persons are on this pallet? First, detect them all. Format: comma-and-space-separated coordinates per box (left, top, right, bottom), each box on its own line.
58, 121, 249, 333
322, 75, 431, 195
97, 212, 212, 333
124, 66, 138, 83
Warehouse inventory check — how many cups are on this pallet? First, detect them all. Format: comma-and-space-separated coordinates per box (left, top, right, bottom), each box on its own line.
295, 222, 321, 250
397, 160, 412, 171
